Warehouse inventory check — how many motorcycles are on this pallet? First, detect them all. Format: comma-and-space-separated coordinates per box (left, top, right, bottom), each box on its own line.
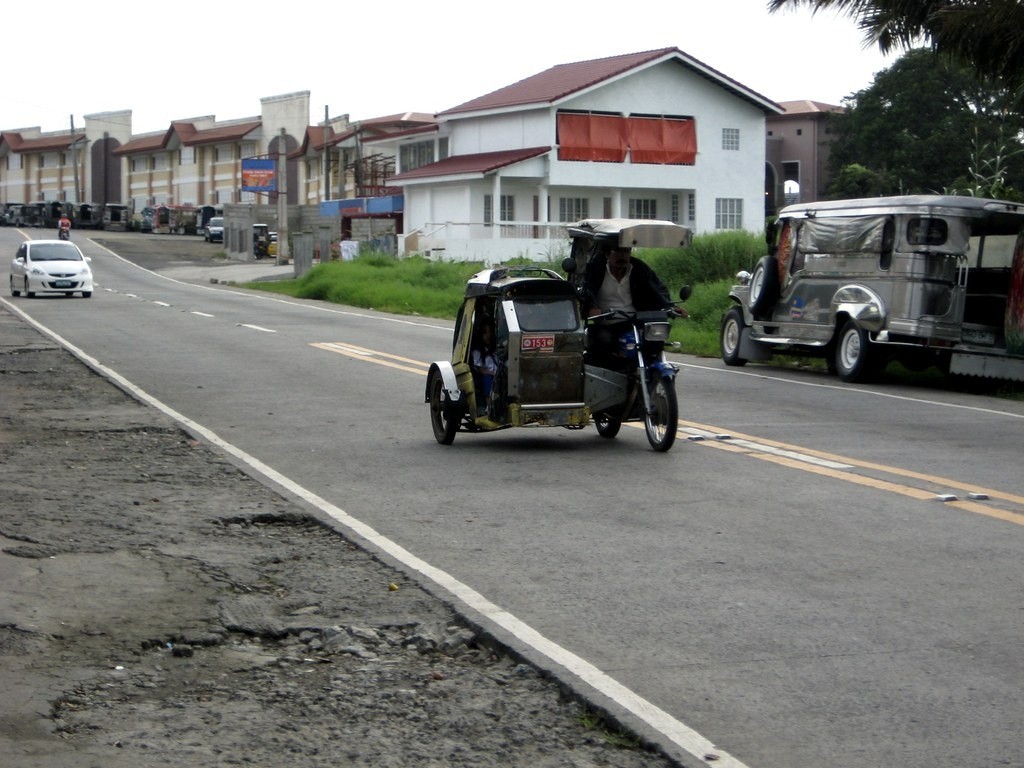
561, 257, 692, 453
58, 223, 70, 240
254, 235, 266, 259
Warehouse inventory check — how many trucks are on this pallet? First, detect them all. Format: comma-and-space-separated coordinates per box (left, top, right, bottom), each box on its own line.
719, 194, 1024, 384
29, 200, 217, 234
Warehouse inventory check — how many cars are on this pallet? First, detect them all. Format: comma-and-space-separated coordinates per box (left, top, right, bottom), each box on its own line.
10, 241, 94, 297
204, 217, 224, 243
267, 232, 278, 257
1, 205, 44, 228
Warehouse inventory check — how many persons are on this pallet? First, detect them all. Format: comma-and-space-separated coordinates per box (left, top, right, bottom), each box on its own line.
58, 213, 71, 238
471, 313, 501, 411
580, 235, 689, 372
